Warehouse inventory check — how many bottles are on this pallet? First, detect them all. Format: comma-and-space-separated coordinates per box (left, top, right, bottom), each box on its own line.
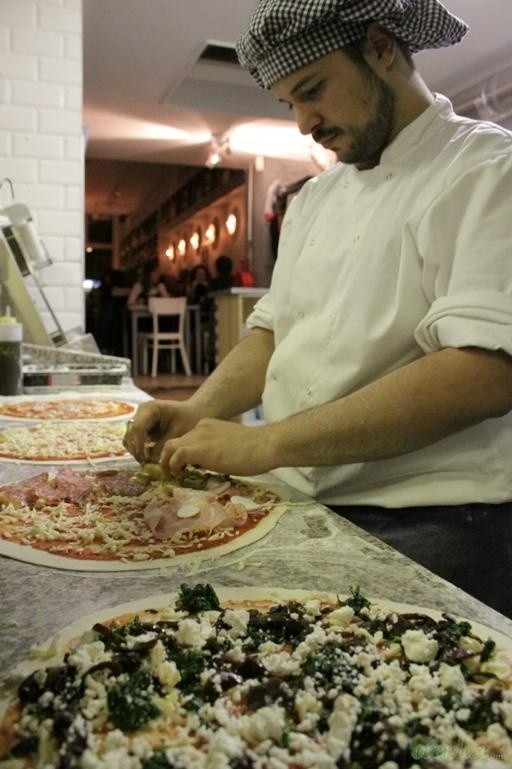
0, 305, 23, 394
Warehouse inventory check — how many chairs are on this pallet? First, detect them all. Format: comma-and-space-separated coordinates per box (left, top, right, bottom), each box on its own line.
138, 296, 193, 378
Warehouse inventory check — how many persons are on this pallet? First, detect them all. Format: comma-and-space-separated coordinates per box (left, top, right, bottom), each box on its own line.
99, 255, 237, 374
123, 2, 511, 614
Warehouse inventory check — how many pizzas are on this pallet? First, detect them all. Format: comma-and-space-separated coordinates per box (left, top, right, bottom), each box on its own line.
0, 398, 288, 571
0, 582, 512, 769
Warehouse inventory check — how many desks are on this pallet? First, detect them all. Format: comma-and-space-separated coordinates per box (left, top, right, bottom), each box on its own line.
128, 304, 202, 377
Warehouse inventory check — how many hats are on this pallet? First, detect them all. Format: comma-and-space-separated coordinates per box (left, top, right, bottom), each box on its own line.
233, 0, 470, 89
